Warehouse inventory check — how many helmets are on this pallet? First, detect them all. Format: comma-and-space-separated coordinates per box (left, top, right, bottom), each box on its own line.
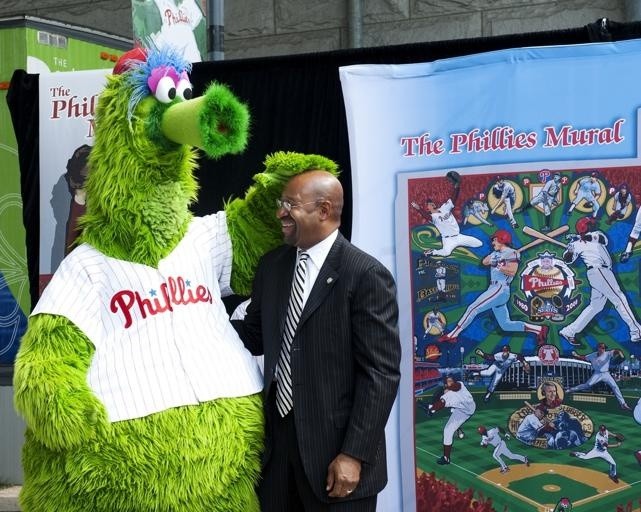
575, 217, 596, 233
599, 425, 606, 431
478, 426, 486, 435
490, 230, 512, 243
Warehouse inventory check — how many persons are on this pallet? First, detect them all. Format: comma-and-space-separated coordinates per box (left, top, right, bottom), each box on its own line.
221, 169, 404, 512
63, 143, 94, 258
412, 171, 641, 484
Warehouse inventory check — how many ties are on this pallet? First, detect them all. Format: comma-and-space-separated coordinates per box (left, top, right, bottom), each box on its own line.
275, 249, 308, 417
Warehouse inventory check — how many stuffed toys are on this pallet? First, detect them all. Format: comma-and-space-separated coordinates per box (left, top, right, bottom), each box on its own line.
13, 29, 343, 512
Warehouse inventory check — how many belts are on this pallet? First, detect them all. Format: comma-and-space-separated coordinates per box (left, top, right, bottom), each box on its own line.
588, 264, 611, 270
490, 280, 510, 285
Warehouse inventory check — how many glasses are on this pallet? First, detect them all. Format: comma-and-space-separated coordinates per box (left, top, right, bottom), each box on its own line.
274, 197, 319, 212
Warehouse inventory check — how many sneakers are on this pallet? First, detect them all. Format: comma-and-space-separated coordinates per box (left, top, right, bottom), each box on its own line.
620, 252, 633, 262
536, 326, 549, 346
609, 474, 619, 483
559, 331, 581, 346
437, 456, 450, 464
500, 467, 511, 473
424, 248, 434, 257
438, 334, 458, 344
524, 457, 529, 467
570, 451, 585, 457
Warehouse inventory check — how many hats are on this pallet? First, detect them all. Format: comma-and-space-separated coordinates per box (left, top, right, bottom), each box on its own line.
599, 343, 607, 349
423, 199, 432, 210
503, 346, 509, 351
536, 405, 548, 415
444, 376, 456, 387
